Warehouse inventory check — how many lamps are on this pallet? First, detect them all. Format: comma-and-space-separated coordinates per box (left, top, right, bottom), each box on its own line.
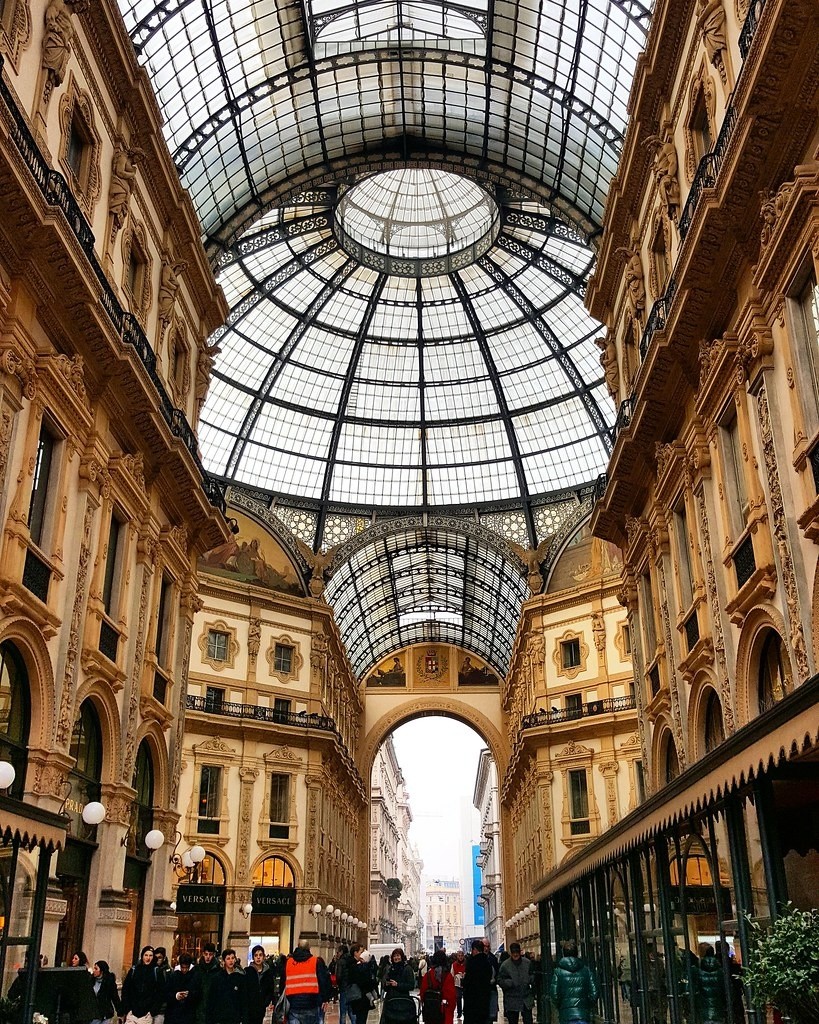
57, 779, 106, 842
239, 904, 253, 919
120, 808, 165, 860
308, 904, 368, 933
0, 760, 16, 790
169, 831, 206, 878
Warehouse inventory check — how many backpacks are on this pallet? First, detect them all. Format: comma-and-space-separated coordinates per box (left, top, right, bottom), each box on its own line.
422, 970, 442, 1024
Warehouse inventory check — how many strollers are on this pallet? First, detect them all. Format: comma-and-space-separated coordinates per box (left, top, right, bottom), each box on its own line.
381, 996, 420, 1024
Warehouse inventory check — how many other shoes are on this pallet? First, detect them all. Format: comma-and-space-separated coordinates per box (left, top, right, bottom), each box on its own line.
457, 1012, 462, 1018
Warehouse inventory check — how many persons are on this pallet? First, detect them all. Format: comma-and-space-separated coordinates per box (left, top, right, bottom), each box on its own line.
159, 258, 190, 328
44, 0, 92, 88
196, 347, 222, 407
110, 147, 146, 228
640, 135, 679, 220
0, 951, 122, 1024
618, 939, 746, 1024
330, 938, 600, 1024
118, 938, 335, 1024
612, 246, 645, 319
695, 0, 728, 70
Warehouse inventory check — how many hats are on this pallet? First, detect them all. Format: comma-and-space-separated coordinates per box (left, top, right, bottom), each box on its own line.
360, 949, 372, 963
141, 946, 154, 955
510, 943, 523, 952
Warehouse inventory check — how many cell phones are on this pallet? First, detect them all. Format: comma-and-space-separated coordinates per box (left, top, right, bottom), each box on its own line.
386, 978, 390, 981
180, 992, 186, 995
